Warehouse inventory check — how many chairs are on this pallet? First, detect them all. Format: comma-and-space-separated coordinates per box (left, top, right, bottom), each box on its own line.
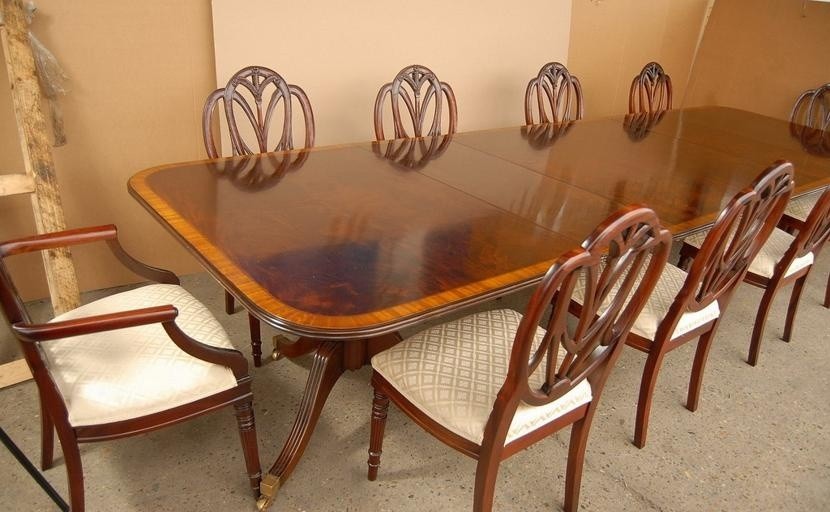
554, 161, 795, 449
374, 63, 459, 138
627, 60, 675, 113
202, 61, 317, 160
371, 207, 676, 508
684, 186, 829, 367
781, 183, 830, 307
523, 63, 585, 124
2, 224, 262, 512
788, 83, 830, 130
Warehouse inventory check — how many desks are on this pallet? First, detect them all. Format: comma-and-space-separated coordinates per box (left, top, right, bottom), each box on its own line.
129, 104, 829, 512
